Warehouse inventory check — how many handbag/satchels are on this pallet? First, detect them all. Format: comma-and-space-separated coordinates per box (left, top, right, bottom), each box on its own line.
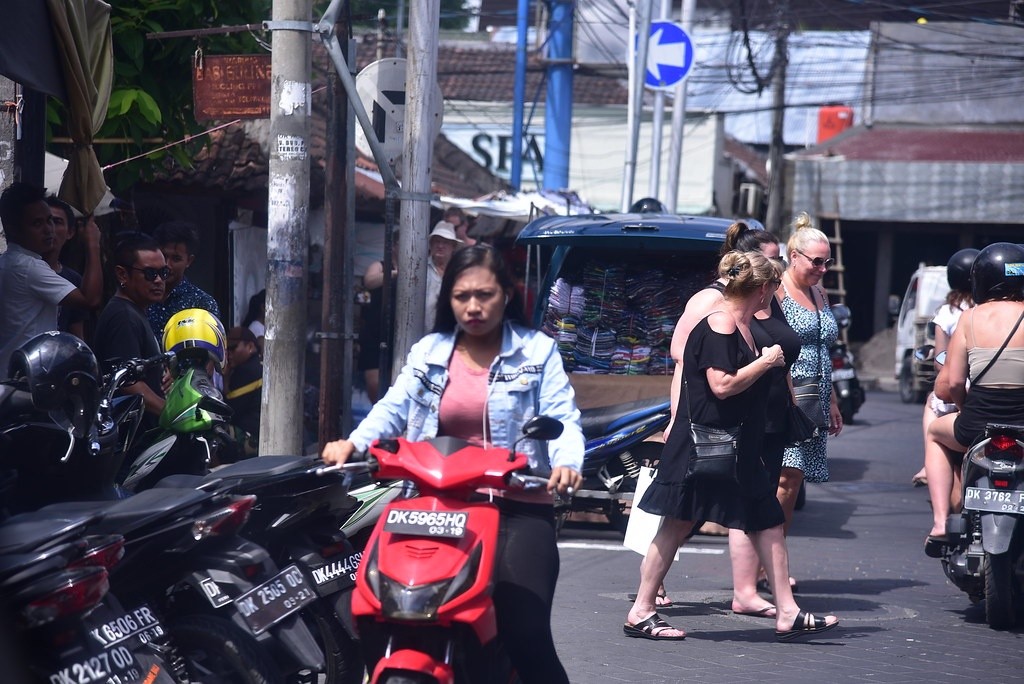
684, 421, 742, 484
788, 376, 828, 429
789, 402, 818, 441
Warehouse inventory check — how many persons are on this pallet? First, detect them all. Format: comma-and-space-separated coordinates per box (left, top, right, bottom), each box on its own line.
923, 242, 1024, 557
242, 291, 265, 356
623, 208, 842, 644
911, 248, 980, 488
318, 239, 586, 683
225, 326, 264, 435
360, 204, 493, 402
145, 223, 224, 351
94, 227, 180, 489
46, 193, 89, 343
949, 453, 964, 514
0, 182, 106, 385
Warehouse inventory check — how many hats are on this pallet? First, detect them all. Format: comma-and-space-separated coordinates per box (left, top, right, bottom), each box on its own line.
429, 220, 464, 243
225, 326, 257, 352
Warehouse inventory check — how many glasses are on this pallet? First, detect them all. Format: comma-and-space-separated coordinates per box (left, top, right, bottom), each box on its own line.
798, 252, 834, 269
768, 256, 783, 261
770, 278, 781, 291
123, 265, 170, 282
453, 221, 466, 230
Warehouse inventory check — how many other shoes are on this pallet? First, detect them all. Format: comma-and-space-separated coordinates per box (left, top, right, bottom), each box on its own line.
756, 575, 796, 595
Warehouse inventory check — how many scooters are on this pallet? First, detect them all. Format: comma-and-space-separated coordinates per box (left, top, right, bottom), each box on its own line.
317, 416, 565, 684
0, 351, 407, 684
560, 395, 672, 536
824, 302, 867, 426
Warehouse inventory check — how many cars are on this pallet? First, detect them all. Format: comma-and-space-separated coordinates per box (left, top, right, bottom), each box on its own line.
893, 262, 951, 406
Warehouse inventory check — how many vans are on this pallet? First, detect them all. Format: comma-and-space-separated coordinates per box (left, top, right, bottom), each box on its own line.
514, 197, 763, 407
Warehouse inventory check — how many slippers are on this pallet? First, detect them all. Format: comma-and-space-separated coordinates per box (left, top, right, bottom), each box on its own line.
733, 605, 778, 619
776, 610, 839, 641
911, 475, 930, 485
623, 612, 686, 640
627, 589, 673, 607
926, 533, 947, 558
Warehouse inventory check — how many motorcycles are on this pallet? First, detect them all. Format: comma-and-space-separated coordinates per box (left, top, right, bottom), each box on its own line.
933, 350, 1024, 632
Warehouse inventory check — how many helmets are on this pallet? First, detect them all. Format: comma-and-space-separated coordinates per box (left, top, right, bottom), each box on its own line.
970, 242, 1024, 304
947, 249, 984, 295
163, 308, 227, 374
9, 329, 98, 413
